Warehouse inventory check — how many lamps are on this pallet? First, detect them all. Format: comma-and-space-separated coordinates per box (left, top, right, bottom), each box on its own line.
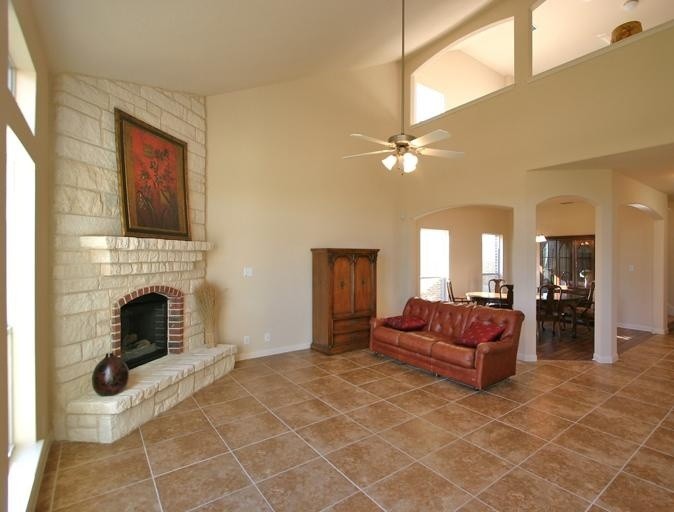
381, 151, 418, 173
341, 0, 463, 173
536, 233, 548, 242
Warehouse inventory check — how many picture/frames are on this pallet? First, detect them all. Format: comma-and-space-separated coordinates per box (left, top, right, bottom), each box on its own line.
114, 107, 191, 240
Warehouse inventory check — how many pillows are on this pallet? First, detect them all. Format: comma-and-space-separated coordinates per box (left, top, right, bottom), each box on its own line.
381, 312, 429, 330
452, 320, 505, 348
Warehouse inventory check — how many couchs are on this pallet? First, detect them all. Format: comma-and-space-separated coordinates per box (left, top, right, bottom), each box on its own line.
369, 297, 524, 390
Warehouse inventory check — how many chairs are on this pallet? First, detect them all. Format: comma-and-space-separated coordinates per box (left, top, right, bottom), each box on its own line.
445, 278, 595, 339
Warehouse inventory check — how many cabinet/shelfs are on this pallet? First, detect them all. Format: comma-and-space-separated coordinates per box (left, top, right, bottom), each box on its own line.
311, 252, 377, 356
